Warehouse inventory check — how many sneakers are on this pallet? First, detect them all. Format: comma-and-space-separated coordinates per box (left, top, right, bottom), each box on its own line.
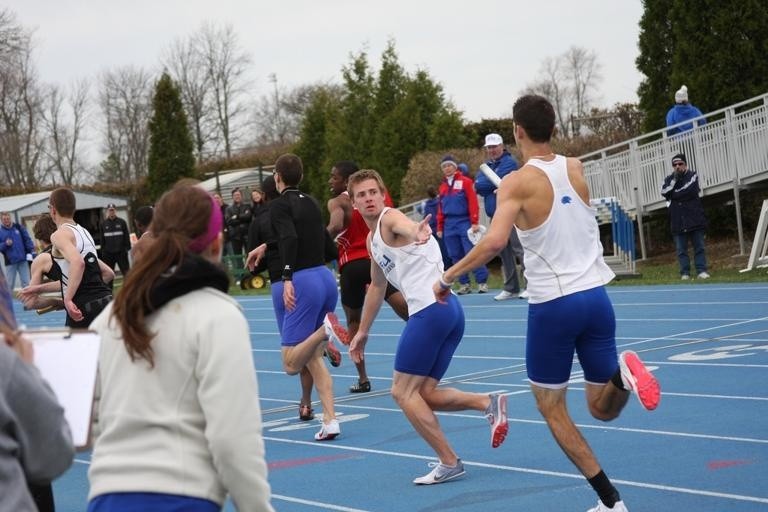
455, 282, 472, 295
494, 289, 513, 302
299, 399, 314, 421
484, 392, 511, 449
518, 289, 529, 299
585, 499, 629, 512
681, 274, 690, 281
477, 283, 489, 294
619, 349, 662, 412
412, 458, 470, 486
697, 271, 711, 281
314, 419, 341, 441
348, 378, 371, 394
322, 311, 351, 368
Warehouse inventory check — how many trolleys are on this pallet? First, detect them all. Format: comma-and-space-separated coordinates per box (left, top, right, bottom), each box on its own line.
221, 253, 269, 290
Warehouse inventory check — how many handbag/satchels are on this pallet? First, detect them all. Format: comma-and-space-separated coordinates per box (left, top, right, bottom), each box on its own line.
13, 223, 32, 264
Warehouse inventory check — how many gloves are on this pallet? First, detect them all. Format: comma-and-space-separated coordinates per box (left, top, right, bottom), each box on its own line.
26, 253, 34, 262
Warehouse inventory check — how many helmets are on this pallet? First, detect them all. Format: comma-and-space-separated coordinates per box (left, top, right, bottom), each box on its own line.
107, 203, 117, 210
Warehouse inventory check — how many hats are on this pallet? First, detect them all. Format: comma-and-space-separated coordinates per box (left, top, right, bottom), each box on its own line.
674, 83, 689, 103
440, 155, 459, 170
483, 133, 504, 147
671, 153, 687, 164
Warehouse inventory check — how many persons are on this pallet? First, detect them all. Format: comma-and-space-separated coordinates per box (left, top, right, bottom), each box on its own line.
661, 153, 710, 281
665, 85, 707, 138
